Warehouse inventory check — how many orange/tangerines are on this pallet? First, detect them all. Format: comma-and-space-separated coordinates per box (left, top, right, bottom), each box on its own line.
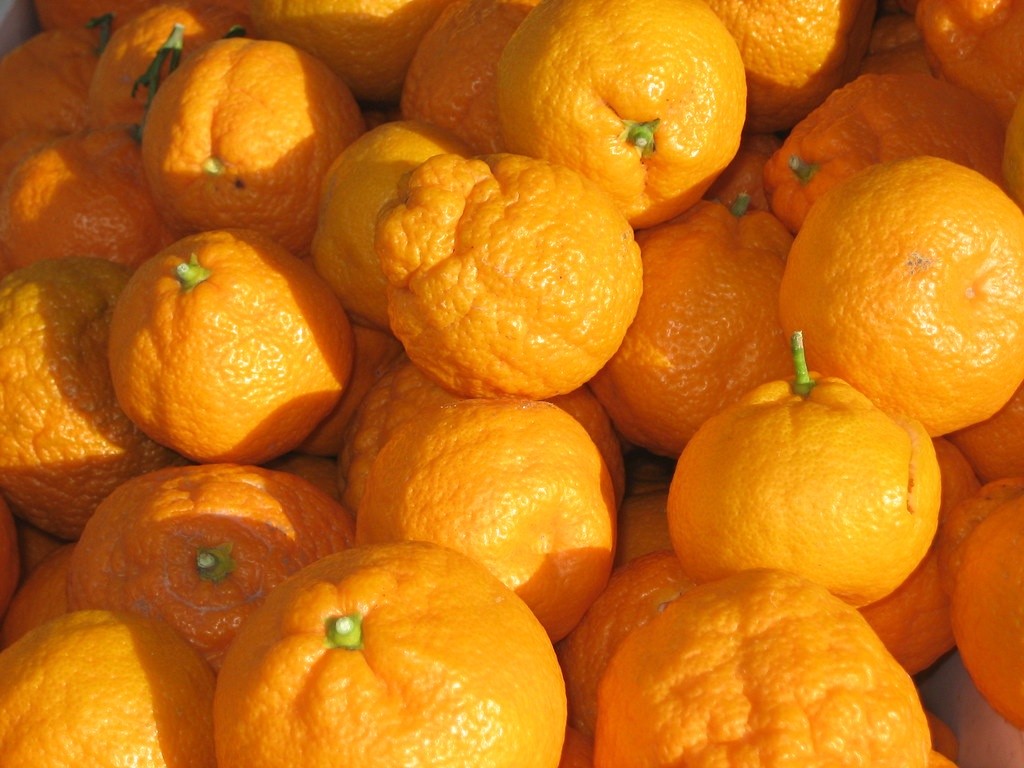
0, 0, 1024, 768
